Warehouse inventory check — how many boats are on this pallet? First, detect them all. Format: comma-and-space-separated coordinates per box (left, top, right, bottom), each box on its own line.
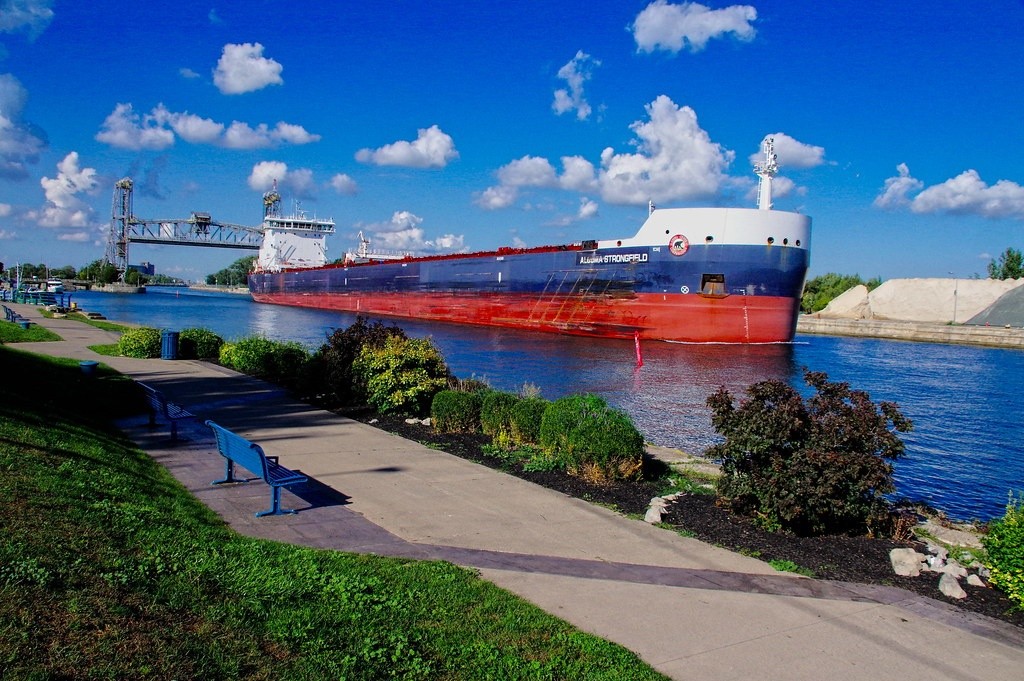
45, 267, 65, 294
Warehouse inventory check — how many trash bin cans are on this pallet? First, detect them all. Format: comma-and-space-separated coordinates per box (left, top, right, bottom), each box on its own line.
162, 330, 179, 361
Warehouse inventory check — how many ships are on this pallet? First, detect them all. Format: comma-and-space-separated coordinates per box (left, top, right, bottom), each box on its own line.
244, 133, 815, 345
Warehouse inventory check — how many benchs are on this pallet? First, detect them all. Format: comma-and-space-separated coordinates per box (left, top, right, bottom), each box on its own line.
2, 305, 16, 320
206, 419, 308, 520
137, 380, 198, 445
6, 307, 21, 323
